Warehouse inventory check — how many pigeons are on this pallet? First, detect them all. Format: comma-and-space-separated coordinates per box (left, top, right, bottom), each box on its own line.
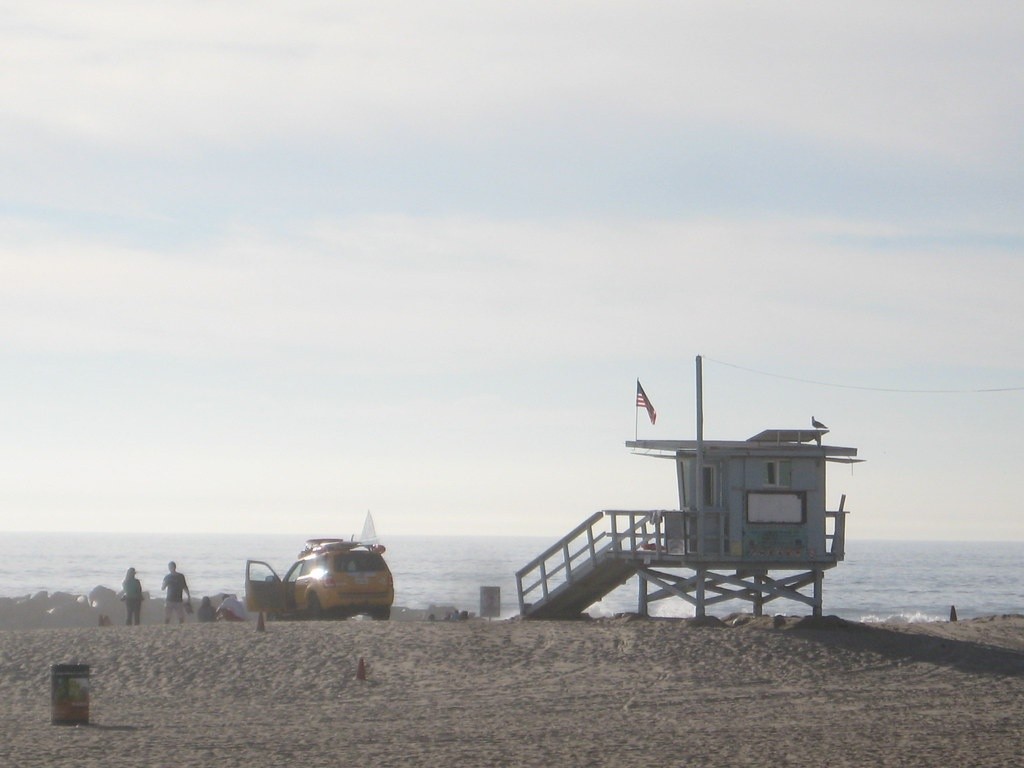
812, 416, 829, 430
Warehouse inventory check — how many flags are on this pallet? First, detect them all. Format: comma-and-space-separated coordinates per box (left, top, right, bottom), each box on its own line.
636, 379, 656, 425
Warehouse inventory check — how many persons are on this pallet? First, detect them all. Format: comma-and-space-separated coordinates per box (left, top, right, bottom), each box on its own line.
162, 561, 190, 624
217, 594, 246, 622
123, 568, 144, 625
198, 597, 217, 622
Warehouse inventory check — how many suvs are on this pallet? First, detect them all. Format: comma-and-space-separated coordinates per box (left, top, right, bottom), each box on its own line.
244, 538, 393, 622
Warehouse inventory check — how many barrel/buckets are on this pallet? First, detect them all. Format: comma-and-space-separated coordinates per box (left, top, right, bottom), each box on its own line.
48, 664, 90, 725
